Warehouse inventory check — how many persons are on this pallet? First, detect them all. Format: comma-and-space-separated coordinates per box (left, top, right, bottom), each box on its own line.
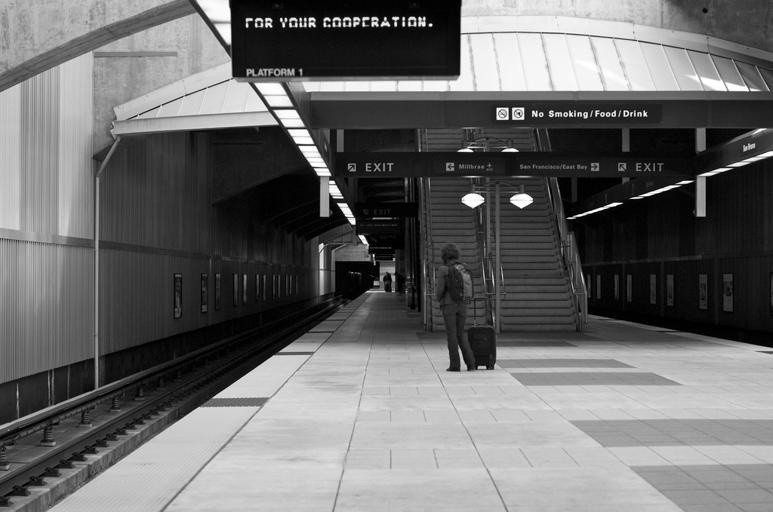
386, 273, 391, 292
383, 272, 387, 285
394, 272, 402, 292
435, 243, 476, 372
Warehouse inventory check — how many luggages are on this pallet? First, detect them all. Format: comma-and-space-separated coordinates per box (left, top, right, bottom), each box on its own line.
469, 297, 496, 370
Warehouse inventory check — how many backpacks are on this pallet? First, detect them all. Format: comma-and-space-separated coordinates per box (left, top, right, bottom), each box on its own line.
448, 261, 474, 301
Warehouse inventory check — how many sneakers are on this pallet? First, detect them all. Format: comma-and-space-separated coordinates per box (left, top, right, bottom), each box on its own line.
447, 367, 460, 371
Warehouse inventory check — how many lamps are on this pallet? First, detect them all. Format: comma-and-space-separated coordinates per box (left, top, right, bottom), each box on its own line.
455, 135, 535, 209
